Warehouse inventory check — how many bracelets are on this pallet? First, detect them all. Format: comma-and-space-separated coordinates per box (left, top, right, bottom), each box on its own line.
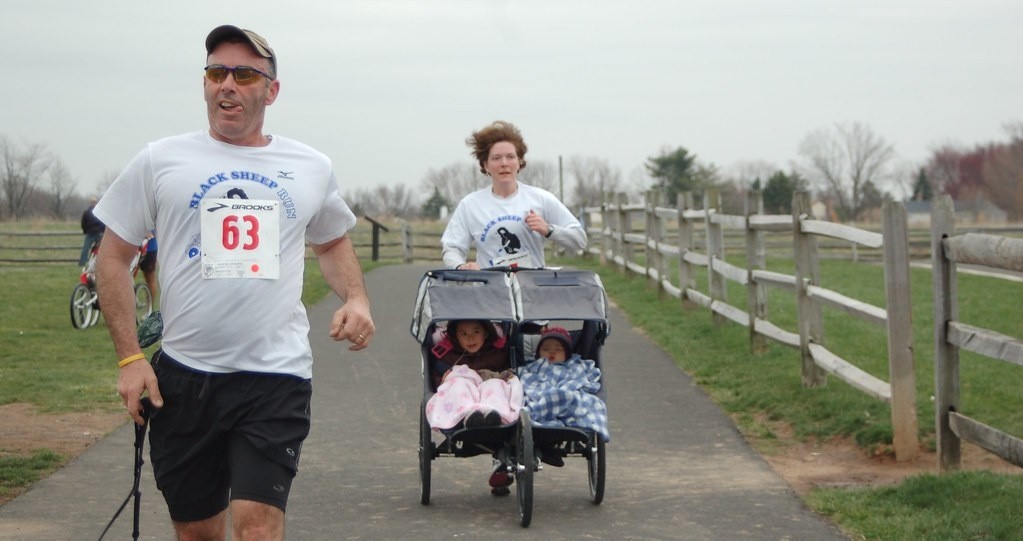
118, 353, 145, 369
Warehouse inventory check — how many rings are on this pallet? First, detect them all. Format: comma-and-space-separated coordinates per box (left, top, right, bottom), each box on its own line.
359, 334, 366, 340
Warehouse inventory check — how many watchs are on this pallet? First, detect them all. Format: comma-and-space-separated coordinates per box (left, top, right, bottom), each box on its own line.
545, 224, 554, 238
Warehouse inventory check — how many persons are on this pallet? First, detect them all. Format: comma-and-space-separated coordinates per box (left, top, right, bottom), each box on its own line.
533, 328, 573, 366
79, 198, 106, 266
140, 230, 157, 322
442, 120, 587, 497
431, 319, 515, 427
93, 25, 376, 541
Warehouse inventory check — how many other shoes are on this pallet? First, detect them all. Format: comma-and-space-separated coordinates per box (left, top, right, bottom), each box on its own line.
140, 311, 152, 322
464, 409, 502, 427
488, 459, 515, 496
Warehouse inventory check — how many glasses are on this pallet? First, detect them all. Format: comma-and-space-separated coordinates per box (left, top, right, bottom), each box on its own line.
205, 63, 273, 85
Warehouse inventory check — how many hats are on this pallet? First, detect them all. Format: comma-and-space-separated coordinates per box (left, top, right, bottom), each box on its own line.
533, 327, 573, 361
204, 24, 277, 80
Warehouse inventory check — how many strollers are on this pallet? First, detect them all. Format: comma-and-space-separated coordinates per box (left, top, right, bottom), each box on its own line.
409, 269, 612, 528
71, 205, 152, 331
